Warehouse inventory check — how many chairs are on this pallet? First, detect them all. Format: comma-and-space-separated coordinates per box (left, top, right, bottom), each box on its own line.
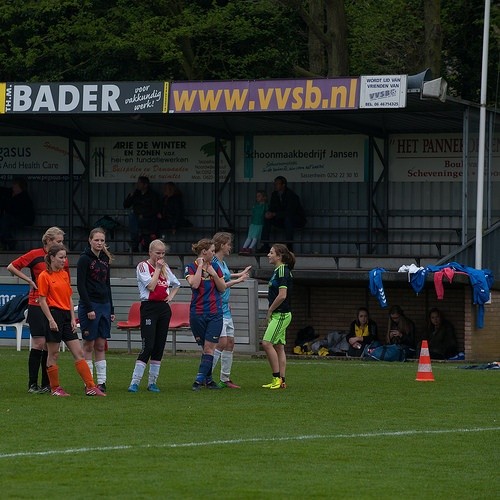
0, 309, 32, 352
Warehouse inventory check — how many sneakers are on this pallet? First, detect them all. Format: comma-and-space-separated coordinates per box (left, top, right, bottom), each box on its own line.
86, 386, 106, 396
97, 383, 106, 391
224, 381, 241, 389
41, 385, 51, 393
51, 386, 70, 396
262, 381, 286, 389
206, 381, 222, 389
147, 383, 160, 392
192, 380, 202, 391
270, 376, 282, 389
128, 384, 138, 392
29, 383, 41, 393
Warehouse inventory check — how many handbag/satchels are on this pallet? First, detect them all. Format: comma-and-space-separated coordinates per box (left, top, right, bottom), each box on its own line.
364, 343, 406, 362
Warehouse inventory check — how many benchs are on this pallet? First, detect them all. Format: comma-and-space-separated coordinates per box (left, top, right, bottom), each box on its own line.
116, 302, 189, 356
0, 225, 461, 269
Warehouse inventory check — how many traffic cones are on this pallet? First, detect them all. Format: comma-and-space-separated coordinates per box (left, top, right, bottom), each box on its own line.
414, 340, 436, 381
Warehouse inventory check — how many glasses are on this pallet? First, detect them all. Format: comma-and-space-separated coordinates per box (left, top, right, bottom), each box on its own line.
391, 316, 399, 319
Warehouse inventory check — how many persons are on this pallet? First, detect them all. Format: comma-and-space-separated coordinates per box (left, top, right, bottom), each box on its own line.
38, 246, 106, 397
184, 239, 226, 390
210, 232, 252, 389
346, 308, 381, 357
239, 190, 270, 254
7, 227, 71, 393
127, 240, 180, 392
371, 305, 417, 362
121, 177, 184, 253
414, 307, 457, 359
77, 227, 117, 392
260, 243, 296, 390
256, 176, 306, 254
0, 177, 34, 249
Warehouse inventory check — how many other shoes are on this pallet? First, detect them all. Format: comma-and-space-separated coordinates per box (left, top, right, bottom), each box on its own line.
4, 244, 10, 250
238, 248, 250, 254
125, 247, 140, 252
